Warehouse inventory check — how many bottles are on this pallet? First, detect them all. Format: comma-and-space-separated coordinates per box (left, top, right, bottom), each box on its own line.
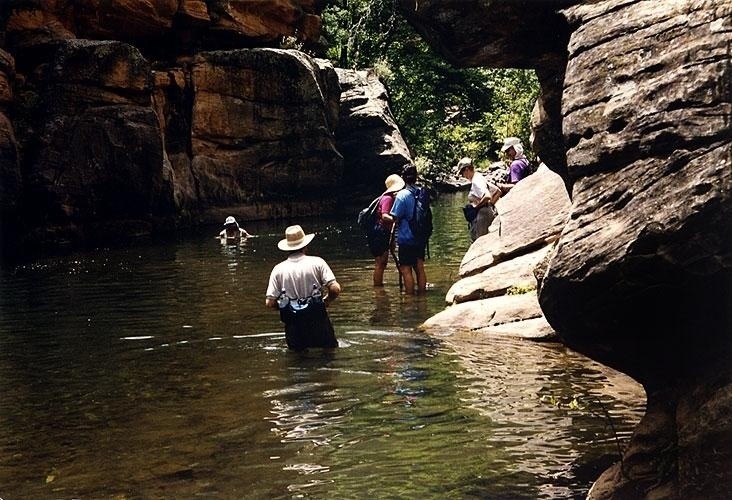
277, 282, 330, 312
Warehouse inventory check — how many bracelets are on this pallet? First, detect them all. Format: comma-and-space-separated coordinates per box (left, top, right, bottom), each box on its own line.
494, 181, 497, 186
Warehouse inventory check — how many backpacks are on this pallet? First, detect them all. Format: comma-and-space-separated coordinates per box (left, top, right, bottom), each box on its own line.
521, 161, 533, 179
402, 186, 433, 259
357, 195, 394, 245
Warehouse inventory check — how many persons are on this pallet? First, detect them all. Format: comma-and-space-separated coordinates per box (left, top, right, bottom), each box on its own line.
368, 174, 405, 285
458, 157, 502, 244
220, 215, 250, 237
389, 163, 429, 289
266, 225, 341, 348
490, 137, 530, 194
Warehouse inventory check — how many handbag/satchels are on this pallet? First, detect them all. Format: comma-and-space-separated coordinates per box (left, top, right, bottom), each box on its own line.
462, 205, 481, 230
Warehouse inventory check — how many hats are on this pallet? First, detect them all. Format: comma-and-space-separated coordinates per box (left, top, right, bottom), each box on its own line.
383, 174, 405, 195
500, 137, 527, 159
401, 164, 417, 175
224, 216, 240, 229
455, 157, 472, 176
277, 225, 315, 251
